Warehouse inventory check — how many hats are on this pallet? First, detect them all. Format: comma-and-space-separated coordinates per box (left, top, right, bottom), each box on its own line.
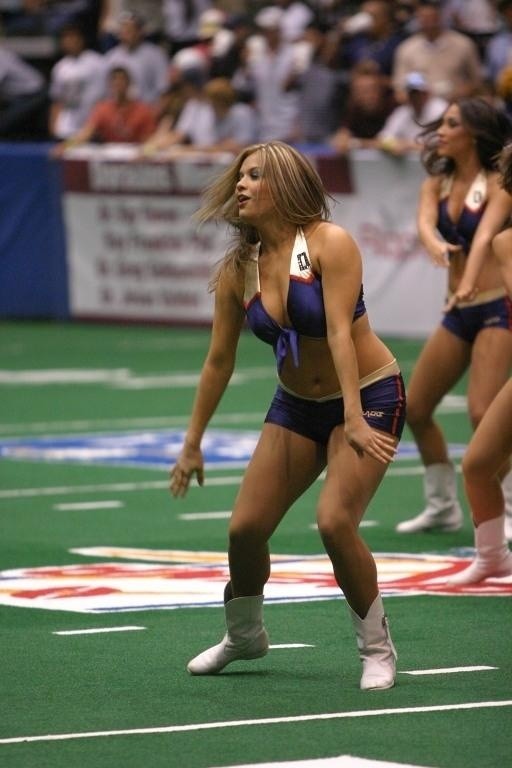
171, 2, 433, 92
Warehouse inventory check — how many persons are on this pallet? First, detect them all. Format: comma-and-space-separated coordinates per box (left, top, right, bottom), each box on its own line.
446, 145, 512, 586
167, 141, 406, 694
394, 96, 512, 535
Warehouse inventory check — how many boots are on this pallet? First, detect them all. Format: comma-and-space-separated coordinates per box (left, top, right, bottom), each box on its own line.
396, 460, 463, 535
447, 514, 512, 587
186, 580, 269, 675
345, 592, 398, 692
